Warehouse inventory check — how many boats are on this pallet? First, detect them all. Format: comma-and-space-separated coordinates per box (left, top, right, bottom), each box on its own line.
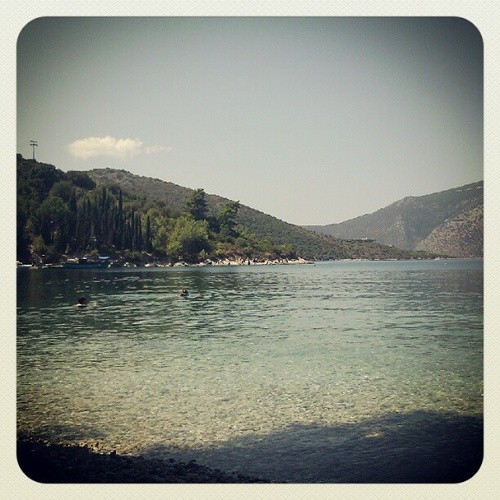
62, 261, 100, 269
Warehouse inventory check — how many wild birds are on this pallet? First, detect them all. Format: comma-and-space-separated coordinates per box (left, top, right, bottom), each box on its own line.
180, 289, 189, 296
72, 292, 98, 308
